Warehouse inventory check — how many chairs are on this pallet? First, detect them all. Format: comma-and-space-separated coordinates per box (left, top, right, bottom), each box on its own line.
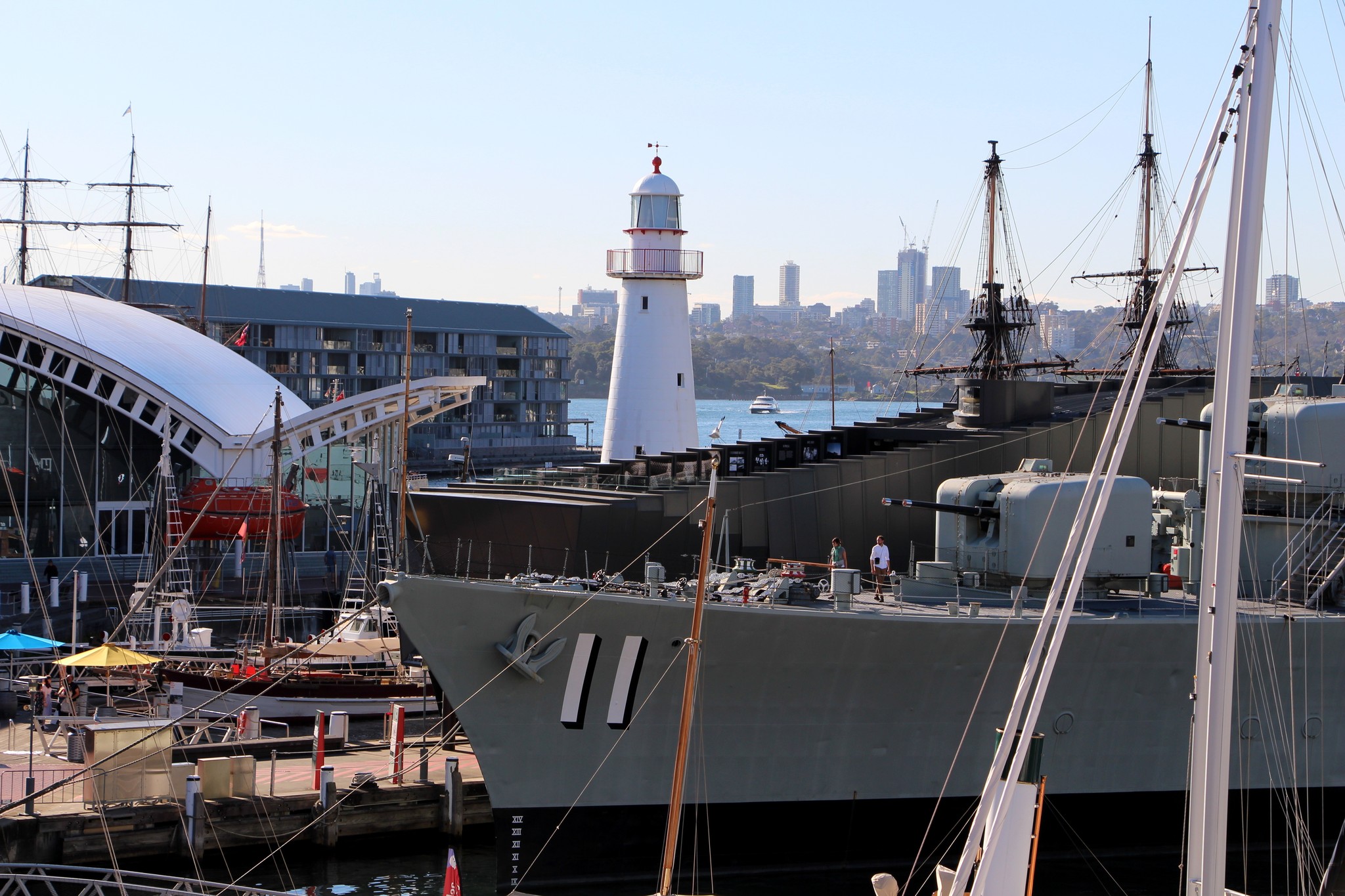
230, 664, 248, 680
245, 666, 261, 682
258, 666, 273, 683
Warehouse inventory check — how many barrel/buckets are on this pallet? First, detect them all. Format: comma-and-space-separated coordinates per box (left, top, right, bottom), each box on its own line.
916, 561, 954, 585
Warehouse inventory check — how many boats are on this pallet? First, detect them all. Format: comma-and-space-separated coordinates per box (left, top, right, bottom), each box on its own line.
748, 387, 781, 414
168, 477, 309, 542
0, 0, 1345, 896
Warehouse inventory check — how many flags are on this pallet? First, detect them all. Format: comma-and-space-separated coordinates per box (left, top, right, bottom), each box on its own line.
335, 389, 344, 402
324, 386, 331, 398
234, 323, 249, 347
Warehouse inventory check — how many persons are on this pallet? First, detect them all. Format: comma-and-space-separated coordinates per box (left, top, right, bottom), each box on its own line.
323, 546, 336, 583
56, 674, 79, 728
43, 560, 58, 584
27, 675, 52, 732
828, 538, 847, 600
407, 470, 420, 480
870, 535, 891, 602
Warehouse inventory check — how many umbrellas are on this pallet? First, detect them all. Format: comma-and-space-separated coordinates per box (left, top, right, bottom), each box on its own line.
0, 630, 66, 691
51, 643, 163, 707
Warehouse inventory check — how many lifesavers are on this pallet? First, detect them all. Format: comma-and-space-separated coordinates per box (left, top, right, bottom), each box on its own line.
237, 710, 247, 734
170, 599, 191, 621
129, 591, 147, 611
131, 663, 151, 681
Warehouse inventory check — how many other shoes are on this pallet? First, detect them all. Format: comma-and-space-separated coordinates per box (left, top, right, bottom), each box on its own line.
41, 724, 48, 729
874, 596, 880, 600
17, 607, 21, 609
15, 599, 21, 602
879, 598, 884, 602
828, 595, 834, 599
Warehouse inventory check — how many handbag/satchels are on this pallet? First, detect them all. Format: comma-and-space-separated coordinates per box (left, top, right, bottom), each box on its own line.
875, 557, 880, 564
56, 703, 61, 711
